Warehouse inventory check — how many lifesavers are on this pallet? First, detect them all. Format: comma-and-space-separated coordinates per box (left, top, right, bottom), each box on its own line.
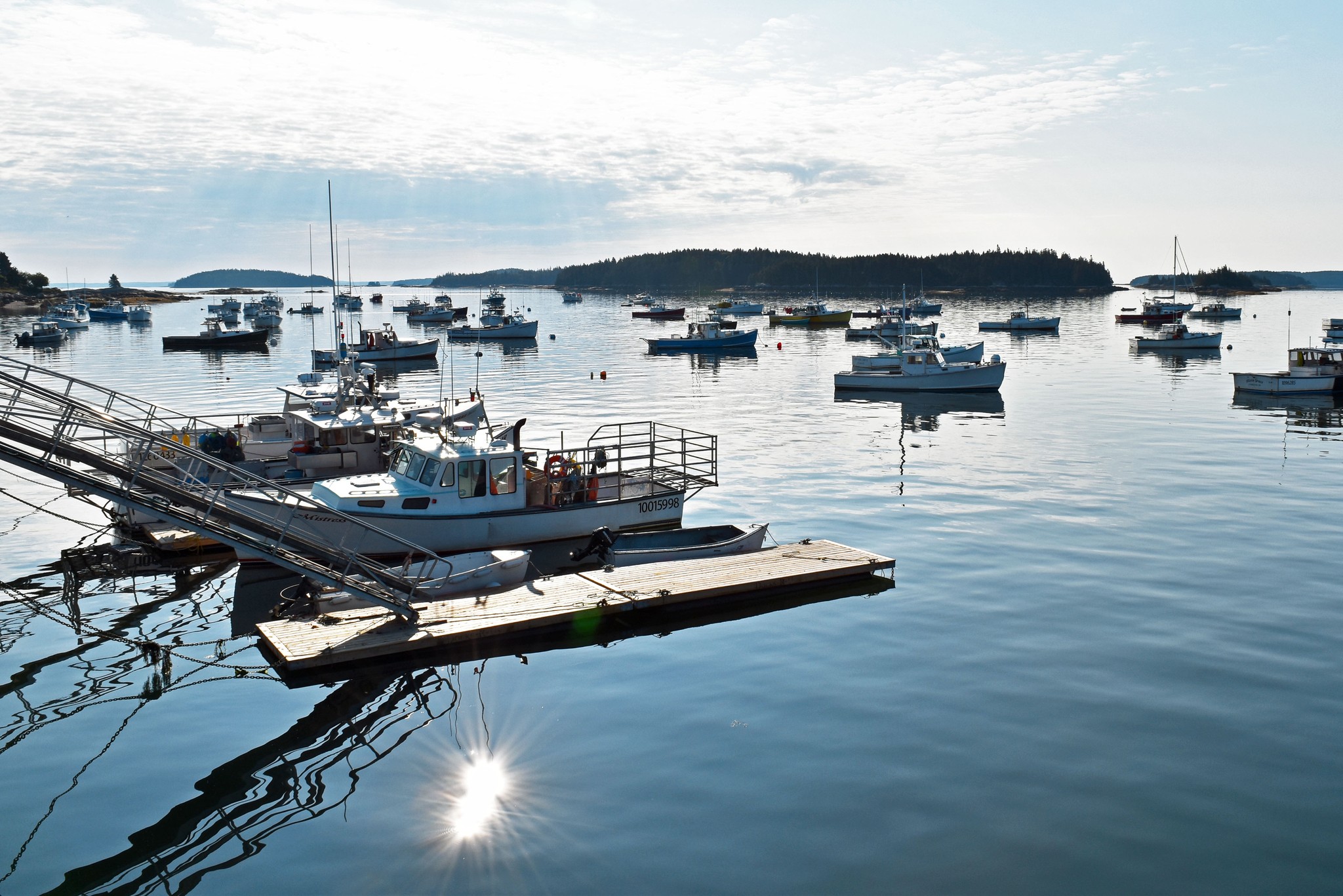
571, 460, 581, 470
1011, 314, 1016, 318
544, 456, 568, 478
366, 334, 369, 340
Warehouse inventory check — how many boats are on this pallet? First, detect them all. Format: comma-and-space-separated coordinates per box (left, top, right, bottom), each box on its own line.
979, 304, 1062, 330
1116, 236, 1242, 324
1128, 318, 1223, 350
1229, 299, 1343, 395
15, 177, 768, 618
771, 264, 1005, 392
